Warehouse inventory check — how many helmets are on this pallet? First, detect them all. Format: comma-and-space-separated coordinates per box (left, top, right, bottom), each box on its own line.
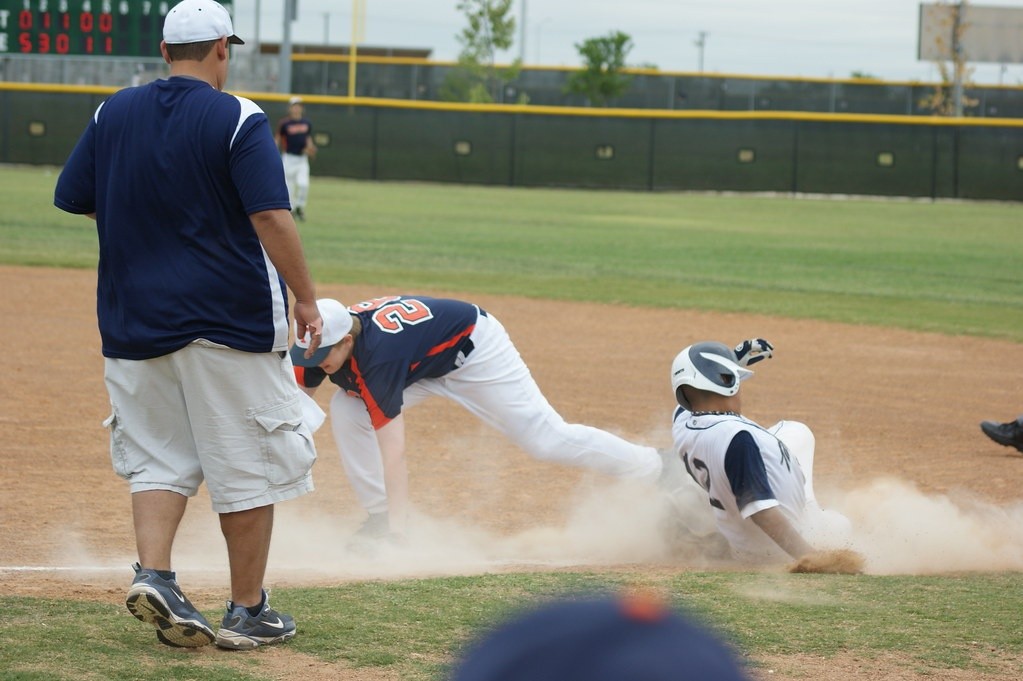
671, 341, 754, 413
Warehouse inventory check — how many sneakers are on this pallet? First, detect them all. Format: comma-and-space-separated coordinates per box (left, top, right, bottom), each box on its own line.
216, 588, 297, 650
125, 562, 215, 647
981, 419, 1023, 453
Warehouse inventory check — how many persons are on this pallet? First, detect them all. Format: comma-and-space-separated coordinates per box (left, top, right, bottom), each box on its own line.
672, 338, 852, 560
274, 96, 316, 222
444, 597, 742, 681
290, 296, 672, 541
980, 415, 1023, 454
54, 0, 323, 650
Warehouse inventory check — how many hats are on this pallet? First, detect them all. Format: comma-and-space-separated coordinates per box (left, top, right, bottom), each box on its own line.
163, 0, 246, 44
454, 590, 741, 681
289, 298, 353, 367
289, 96, 302, 106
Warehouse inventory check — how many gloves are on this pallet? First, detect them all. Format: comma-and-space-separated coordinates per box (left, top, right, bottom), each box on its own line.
734, 338, 774, 366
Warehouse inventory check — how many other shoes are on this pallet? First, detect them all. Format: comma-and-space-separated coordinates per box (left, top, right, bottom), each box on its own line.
296, 207, 305, 219
